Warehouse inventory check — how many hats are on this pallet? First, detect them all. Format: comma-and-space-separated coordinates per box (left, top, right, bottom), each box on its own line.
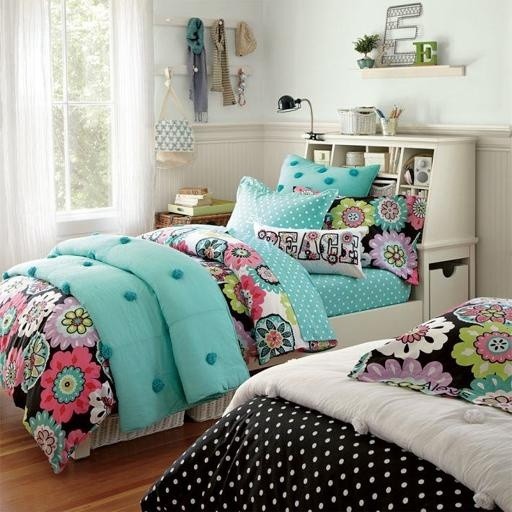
236, 22, 257, 56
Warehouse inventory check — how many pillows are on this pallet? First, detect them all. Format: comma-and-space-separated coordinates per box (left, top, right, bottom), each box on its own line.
348, 296, 512, 415
223, 153, 427, 288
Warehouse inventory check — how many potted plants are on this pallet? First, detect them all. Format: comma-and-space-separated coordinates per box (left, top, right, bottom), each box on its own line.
352, 35, 382, 69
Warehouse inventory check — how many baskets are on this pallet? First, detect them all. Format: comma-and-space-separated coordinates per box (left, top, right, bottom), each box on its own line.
188, 390, 234, 422
338, 111, 376, 135
91, 410, 184, 449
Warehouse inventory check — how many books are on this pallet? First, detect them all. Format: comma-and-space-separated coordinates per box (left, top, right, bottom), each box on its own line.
168, 194, 236, 217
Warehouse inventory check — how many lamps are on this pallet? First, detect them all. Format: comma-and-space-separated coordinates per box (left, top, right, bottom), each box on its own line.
276, 95, 326, 140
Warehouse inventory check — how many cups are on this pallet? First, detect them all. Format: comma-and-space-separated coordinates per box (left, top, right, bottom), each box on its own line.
381, 118, 396, 136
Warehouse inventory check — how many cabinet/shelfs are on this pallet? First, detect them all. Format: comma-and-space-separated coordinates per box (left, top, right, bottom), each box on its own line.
301, 131, 478, 246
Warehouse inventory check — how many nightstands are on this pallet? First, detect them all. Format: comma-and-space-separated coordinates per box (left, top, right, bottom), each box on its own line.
154, 211, 232, 231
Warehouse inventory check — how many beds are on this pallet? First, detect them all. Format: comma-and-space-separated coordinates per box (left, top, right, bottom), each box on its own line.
138, 338, 512, 512
0, 225, 478, 474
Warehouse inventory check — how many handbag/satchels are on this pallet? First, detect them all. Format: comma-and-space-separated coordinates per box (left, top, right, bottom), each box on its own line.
155, 118, 194, 165
